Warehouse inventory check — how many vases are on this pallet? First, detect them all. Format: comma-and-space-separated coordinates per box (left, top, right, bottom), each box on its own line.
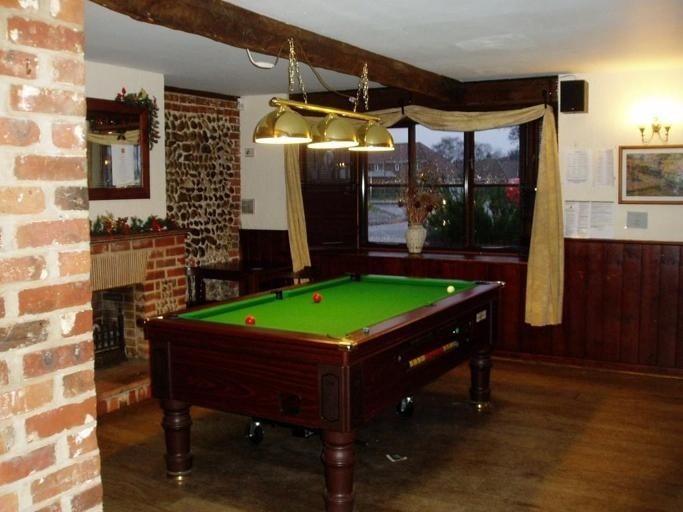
404, 223, 426, 253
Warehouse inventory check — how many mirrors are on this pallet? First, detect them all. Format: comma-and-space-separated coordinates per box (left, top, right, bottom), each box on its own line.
86, 97, 152, 200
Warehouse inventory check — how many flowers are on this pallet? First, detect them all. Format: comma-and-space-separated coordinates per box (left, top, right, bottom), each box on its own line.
391, 169, 444, 224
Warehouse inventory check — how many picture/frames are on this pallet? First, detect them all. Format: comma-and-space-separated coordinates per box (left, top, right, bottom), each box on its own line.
617, 145, 683, 206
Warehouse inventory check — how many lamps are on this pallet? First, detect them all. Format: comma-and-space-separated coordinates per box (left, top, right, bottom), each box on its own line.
249, 36, 396, 155
624, 96, 682, 144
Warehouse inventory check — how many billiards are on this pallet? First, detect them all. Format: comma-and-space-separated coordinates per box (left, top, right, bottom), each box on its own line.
446, 285, 454, 294
312, 292, 320, 302
245, 314, 254, 324
408, 341, 458, 368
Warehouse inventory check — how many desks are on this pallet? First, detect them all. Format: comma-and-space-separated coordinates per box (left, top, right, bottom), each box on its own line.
190, 256, 293, 300
135, 268, 504, 512
